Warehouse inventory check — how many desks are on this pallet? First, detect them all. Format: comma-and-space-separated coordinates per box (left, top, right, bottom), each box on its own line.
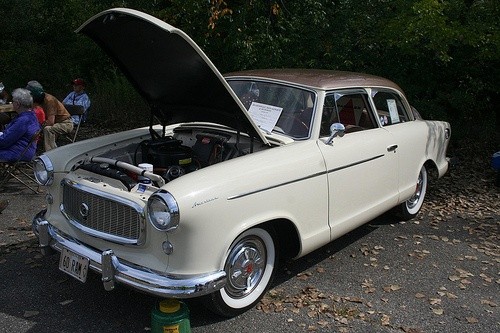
0, 104, 20, 121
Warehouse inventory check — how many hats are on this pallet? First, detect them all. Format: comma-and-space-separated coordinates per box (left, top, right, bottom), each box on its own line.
71, 79, 82, 85
26, 80, 43, 95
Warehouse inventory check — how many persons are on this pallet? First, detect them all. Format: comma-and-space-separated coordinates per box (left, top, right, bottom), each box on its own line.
0, 89, 40, 163
0, 88, 13, 123
26, 81, 74, 151
55, 79, 91, 143
0, 87, 46, 176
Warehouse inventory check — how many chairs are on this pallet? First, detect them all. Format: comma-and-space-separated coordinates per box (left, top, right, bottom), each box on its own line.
0, 120, 46, 195
59, 103, 85, 143
293, 93, 397, 139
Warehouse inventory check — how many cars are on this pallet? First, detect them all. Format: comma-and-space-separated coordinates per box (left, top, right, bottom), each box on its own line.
30, 6, 453, 320
